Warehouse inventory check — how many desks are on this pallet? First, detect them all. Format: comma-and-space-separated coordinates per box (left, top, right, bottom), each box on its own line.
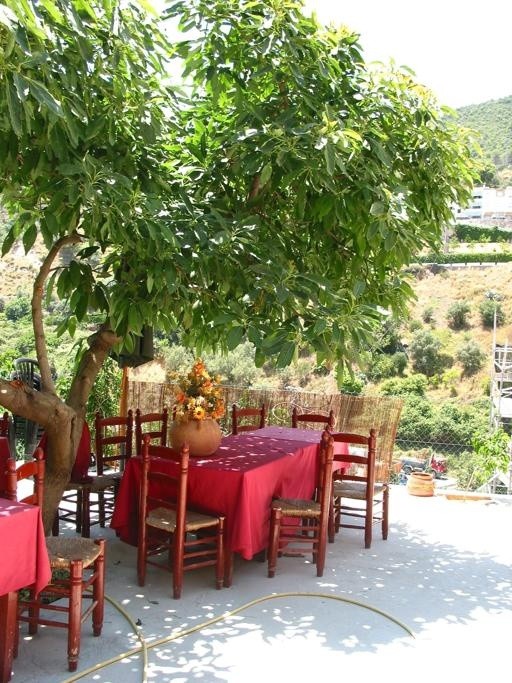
107, 424, 350, 587
1, 493, 54, 679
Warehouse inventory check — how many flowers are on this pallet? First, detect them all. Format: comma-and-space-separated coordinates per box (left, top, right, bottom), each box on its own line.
163, 353, 229, 421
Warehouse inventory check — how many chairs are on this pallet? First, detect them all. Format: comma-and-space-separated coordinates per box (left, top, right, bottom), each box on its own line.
94, 411, 134, 529
233, 403, 268, 436
139, 435, 228, 600
266, 431, 335, 578
133, 407, 167, 453
8, 447, 106, 674
33, 417, 92, 538
290, 408, 335, 431
11, 357, 58, 463
322, 427, 390, 548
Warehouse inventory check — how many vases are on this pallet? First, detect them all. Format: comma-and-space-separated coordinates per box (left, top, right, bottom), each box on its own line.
407, 471, 435, 497
165, 410, 224, 455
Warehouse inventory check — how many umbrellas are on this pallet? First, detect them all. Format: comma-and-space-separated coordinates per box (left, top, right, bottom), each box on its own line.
109, 211, 157, 473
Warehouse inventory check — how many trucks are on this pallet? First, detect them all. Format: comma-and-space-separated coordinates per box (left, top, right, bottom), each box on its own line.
401, 452, 447, 478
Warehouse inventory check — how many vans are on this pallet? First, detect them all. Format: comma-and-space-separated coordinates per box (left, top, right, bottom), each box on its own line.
491, 215, 508, 220
